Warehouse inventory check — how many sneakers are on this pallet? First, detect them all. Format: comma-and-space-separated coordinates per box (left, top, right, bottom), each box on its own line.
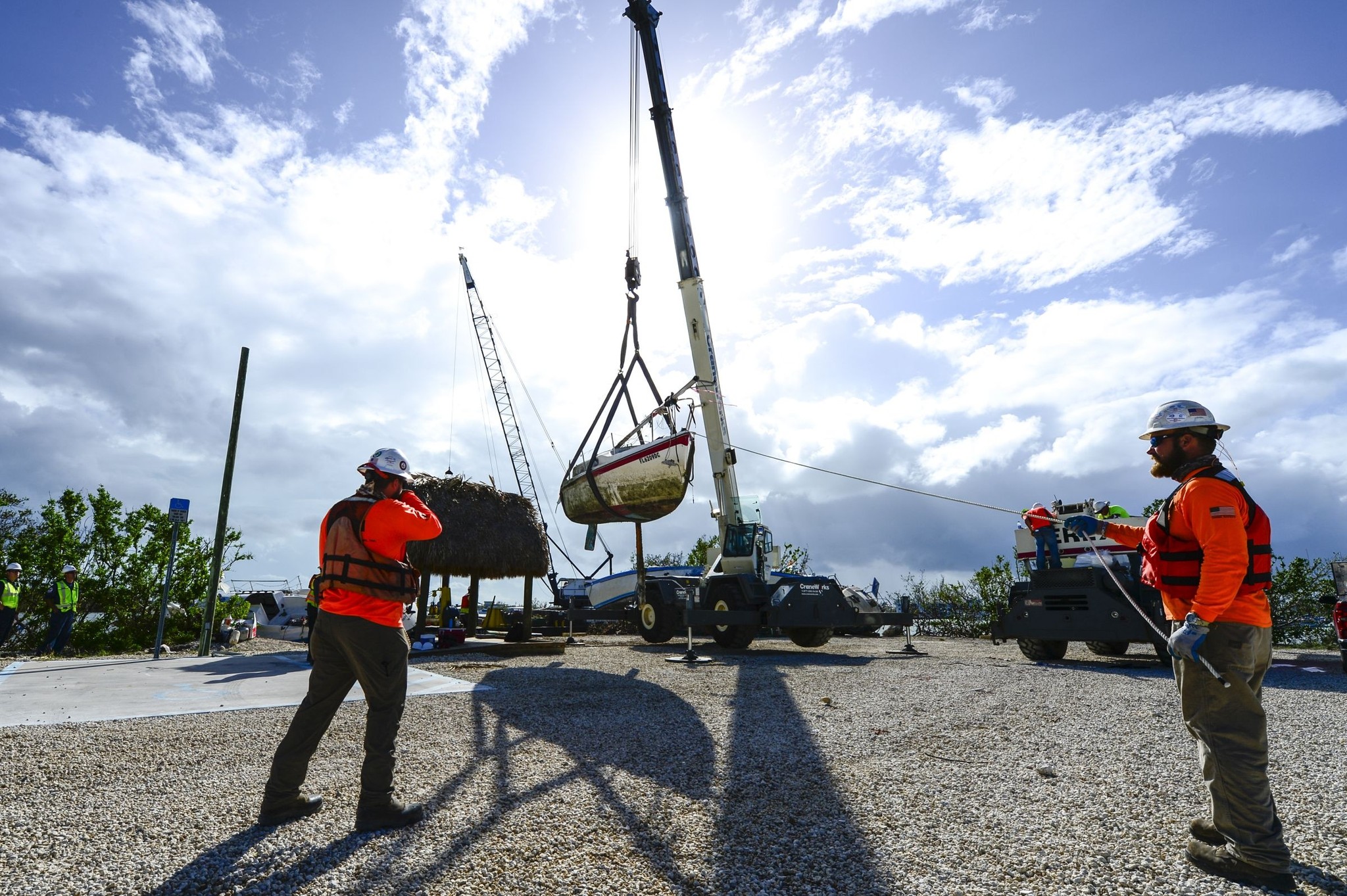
355, 798, 423, 828
258, 794, 323, 826
1185, 841, 1297, 890
1189, 818, 1228, 845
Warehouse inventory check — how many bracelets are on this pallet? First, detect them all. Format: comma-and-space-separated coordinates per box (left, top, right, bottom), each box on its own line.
1102, 522, 1108, 536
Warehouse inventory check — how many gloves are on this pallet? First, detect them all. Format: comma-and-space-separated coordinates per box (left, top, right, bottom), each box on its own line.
1064, 515, 1104, 537
1167, 622, 1209, 662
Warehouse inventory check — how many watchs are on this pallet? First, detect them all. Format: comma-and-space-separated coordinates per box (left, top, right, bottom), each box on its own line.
1185, 612, 1209, 627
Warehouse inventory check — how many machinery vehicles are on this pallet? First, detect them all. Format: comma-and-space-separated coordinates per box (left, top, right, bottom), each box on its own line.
977, 493, 1176, 669
631, 1, 919, 652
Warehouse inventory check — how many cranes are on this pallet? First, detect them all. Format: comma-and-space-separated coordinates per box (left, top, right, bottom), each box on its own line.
457, 252, 613, 636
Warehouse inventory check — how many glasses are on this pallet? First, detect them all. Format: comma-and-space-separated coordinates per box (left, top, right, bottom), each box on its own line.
393, 478, 407, 489
1150, 433, 1207, 448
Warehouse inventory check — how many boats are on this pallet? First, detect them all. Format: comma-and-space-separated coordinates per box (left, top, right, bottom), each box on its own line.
256, 620, 310, 642
558, 398, 696, 526
583, 564, 705, 611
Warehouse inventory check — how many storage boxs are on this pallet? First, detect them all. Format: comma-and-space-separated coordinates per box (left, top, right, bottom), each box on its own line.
420, 634, 436, 647
438, 628, 467, 643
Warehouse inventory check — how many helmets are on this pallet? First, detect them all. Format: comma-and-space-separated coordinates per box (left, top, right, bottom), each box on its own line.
1031, 503, 1046, 510
1093, 501, 1108, 512
357, 448, 414, 482
62, 565, 76, 573
1139, 400, 1230, 440
6, 562, 23, 570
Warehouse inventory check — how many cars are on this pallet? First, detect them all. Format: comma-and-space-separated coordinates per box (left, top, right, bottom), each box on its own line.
1318, 561, 1347, 674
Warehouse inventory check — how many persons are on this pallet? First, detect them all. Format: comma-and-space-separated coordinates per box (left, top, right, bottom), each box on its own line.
0, 563, 22, 648
459, 586, 479, 628
1021, 503, 1062, 569
304, 566, 322, 661
258, 448, 443, 831
437, 598, 441, 616
1093, 501, 1129, 520
35, 565, 79, 657
1064, 400, 1296, 890
